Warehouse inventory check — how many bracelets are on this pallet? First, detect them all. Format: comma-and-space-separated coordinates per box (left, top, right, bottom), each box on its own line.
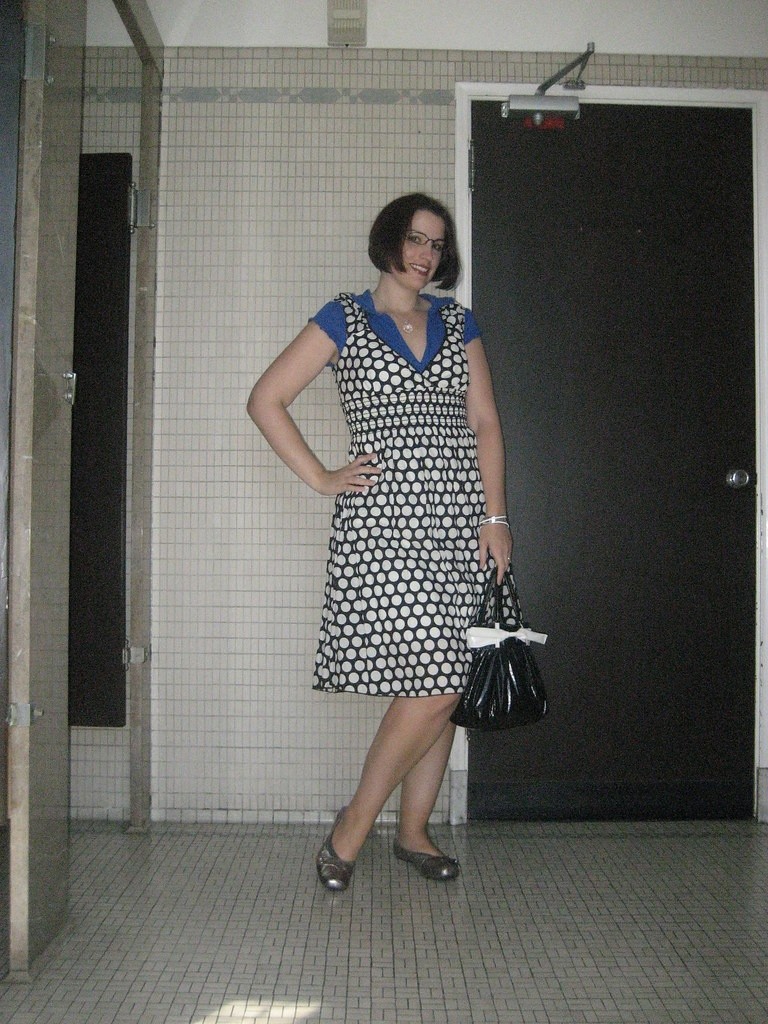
479, 515, 510, 529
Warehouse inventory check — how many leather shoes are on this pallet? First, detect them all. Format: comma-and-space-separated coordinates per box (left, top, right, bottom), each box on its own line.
393, 843, 462, 880
315, 806, 356, 891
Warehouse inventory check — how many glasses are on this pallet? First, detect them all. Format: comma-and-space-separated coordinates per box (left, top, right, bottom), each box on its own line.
404, 230, 445, 252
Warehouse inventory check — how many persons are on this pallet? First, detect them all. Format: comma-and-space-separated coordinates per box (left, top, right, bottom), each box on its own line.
245, 191, 512, 892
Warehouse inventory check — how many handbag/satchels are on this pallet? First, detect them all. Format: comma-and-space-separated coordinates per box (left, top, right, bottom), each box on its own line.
451, 565, 549, 729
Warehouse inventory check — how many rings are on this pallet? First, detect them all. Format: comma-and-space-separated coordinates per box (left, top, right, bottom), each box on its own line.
507, 557, 511, 561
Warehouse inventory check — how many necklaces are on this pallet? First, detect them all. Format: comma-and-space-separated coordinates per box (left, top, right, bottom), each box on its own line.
372, 292, 419, 334
